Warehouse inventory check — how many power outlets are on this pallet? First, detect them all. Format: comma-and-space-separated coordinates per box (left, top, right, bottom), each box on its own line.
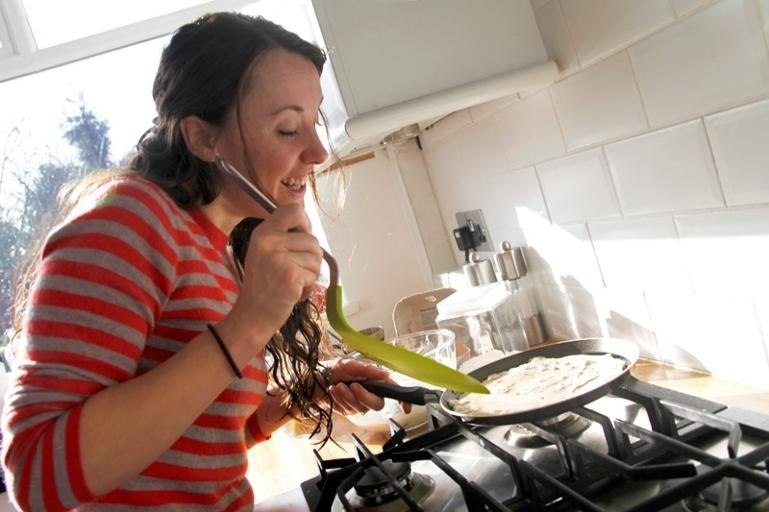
453, 210, 495, 254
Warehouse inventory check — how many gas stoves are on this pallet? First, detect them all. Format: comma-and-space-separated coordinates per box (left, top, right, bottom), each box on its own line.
301, 374, 769, 512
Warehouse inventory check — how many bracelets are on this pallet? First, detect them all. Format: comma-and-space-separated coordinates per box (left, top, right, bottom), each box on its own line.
206, 323, 245, 381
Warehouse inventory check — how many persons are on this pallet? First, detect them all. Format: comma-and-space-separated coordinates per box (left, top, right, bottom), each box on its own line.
0, 14, 414, 512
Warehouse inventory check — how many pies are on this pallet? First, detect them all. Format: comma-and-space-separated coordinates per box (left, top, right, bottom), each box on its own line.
446, 350, 627, 415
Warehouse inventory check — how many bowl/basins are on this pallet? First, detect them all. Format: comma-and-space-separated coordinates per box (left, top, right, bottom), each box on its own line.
332, 329, 456, 434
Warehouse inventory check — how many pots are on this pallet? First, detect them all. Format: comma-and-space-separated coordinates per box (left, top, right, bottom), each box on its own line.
338, 337, 641, 428
